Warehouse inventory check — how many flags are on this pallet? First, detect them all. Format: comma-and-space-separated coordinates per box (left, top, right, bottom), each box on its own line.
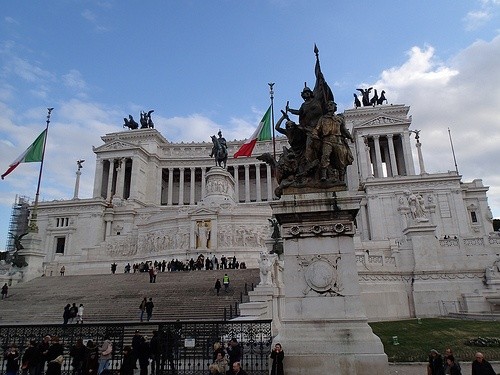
1, 127, 48, 180
234, 102, 273, 159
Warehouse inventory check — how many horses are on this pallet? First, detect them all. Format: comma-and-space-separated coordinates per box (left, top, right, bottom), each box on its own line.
210, 134, 228, 169
122, 112, 154, 130
353, 88, 387, 109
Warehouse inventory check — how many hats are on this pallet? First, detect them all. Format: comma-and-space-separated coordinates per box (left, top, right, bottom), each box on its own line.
135, 329, 140, 333
50, 337, 61, 341
122, 346, 132, 351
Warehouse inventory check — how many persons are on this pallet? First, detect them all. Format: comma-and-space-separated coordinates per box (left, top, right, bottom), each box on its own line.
123, 254, 239, 274
208, 131, 228, 157
120, 346, 136, 375
68, 339, 86, 375
270, 343, 285, 375
212, 337, 249, 375
62, 303, 84, 325
138, 297, 155, 322
214, 273, 230, 296
275, 114, 309, 185
149, 266, 157, 284
428, 347, 497, 375
60, 265, 65, 276
1, 283, 8, 299
95, 334, 113, 375
314, 100, 355, 182
285, 58, 335, 130
4, 334, 64, 375
148, 327, 180, 375
82, 340, 99, 375
131, 329, 145, 369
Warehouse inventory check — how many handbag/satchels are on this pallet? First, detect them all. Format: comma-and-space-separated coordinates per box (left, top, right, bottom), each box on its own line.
101, 342, 112, 356
139, 301, 144, 309
